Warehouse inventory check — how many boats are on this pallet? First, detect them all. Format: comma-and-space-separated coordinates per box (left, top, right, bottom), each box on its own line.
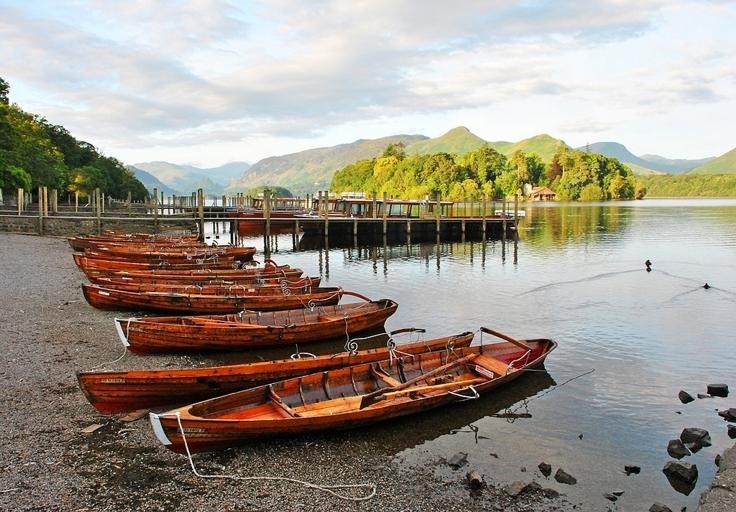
293, 198, 519, 237
230, 192, 365, 231
